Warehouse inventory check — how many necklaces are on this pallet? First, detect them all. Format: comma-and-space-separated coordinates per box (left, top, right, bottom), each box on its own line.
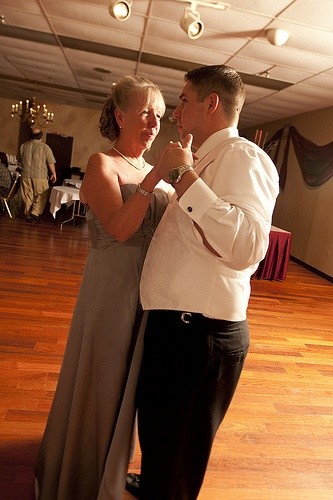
112, 146, 146, 170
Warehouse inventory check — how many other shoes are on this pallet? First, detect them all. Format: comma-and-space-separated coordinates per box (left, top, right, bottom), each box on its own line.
126, 473, 142, 497
30, 213, 41, 222
26, 217, 33, 223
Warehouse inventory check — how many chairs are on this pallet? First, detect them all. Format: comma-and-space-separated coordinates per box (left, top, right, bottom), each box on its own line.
0, 175, 22, 218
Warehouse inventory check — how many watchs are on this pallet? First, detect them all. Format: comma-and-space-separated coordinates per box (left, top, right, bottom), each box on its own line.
168, 164, 193, 186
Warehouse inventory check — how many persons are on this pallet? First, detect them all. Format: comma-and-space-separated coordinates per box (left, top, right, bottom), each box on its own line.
126, 64, 279, 500
16, 128, 57, 222
34, 75, 198, 500
0, 151, 17, 212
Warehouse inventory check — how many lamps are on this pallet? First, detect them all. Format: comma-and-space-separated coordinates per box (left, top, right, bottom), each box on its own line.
267, 29, 289, 46
175, 0, 229, 40
108, 0, 133, 22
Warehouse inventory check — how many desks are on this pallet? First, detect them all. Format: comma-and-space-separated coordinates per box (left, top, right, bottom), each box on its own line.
62, 179, 83, 188
251, 224, 292, 280
50, 186, 86, 231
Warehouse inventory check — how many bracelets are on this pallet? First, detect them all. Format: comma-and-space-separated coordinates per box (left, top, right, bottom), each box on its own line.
136, 182, 154, 197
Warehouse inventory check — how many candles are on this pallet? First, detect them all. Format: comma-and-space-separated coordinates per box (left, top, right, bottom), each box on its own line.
20, 101, 22, 110
37, 105, 40, 112
11, 104, 15, 111
16, 104, 19, 112
30, 108, 33, 114
43, 104, 54, 120
26, 100, 29, 109
